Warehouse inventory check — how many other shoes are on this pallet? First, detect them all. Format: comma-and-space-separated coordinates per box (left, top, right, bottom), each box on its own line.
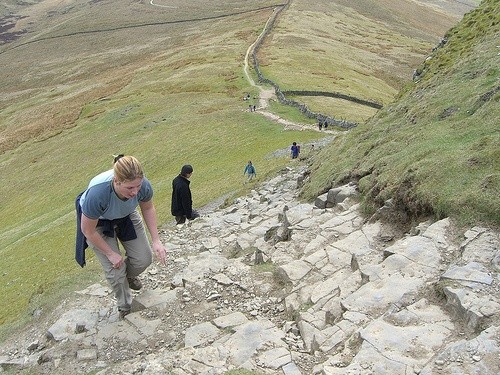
128, 276, 142, 290
119, 310, 130, 318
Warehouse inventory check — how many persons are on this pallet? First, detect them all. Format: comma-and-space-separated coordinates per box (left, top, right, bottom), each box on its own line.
250, 105, 256, 112
74, 154, 167, 318
324, 120, 328, 130
310, 145, 316, 150
243, 160, 256, 179
319, 119, 322, 130
171, 164, 200, 225
289, 142, 299, 159
